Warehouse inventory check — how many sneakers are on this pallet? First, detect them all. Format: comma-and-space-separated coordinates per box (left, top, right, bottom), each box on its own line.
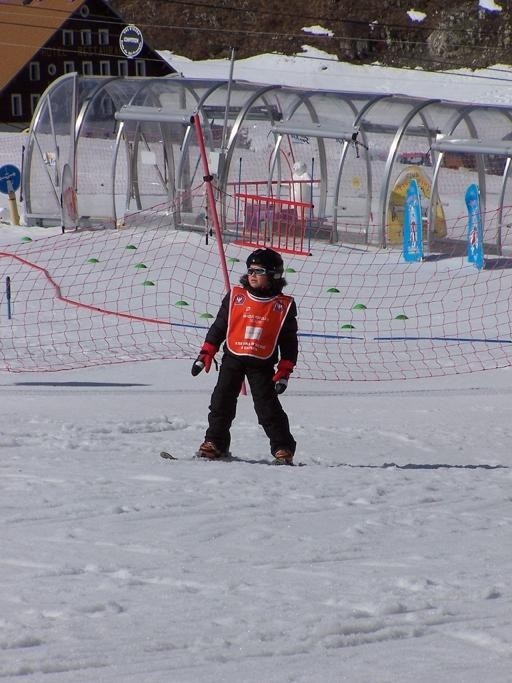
274, 448, 294, 462
199, 440, 230, 455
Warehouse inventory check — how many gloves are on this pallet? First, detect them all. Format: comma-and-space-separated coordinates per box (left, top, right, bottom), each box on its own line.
191, 342, 217, 378
273, 359, 296, 394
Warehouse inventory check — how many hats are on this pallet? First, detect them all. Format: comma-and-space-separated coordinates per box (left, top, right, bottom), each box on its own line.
245, 247, 284, 268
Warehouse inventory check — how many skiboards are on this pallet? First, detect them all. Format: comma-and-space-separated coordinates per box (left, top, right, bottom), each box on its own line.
162, 451, 294, 466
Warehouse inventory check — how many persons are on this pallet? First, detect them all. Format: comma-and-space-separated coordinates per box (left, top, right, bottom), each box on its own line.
192, 246, 301, 462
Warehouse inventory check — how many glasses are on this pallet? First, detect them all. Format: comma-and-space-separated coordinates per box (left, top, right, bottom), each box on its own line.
247, 268, 279, 275
